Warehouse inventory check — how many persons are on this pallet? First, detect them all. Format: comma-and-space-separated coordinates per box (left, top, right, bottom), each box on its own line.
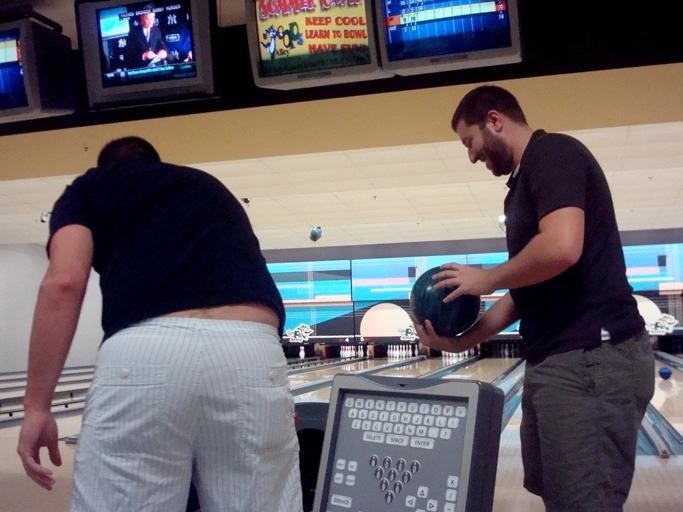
14, 133, 308, 512
123, 4, 168, 67
407, 81, 654, 511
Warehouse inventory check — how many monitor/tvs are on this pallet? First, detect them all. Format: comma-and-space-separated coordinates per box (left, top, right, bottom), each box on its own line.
0, 20, 77, 125
74, 1, 221, 113
244, 0, 396, 90
375, 0, 522, 79
